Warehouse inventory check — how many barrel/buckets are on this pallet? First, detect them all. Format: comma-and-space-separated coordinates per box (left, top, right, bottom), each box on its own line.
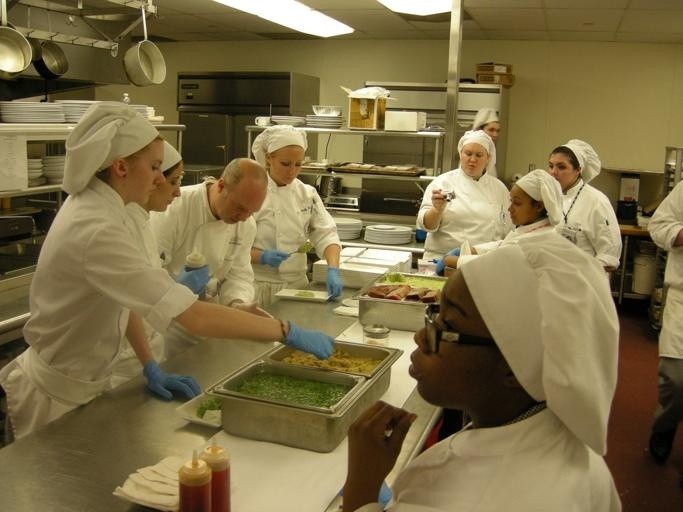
629, 253, 657, 296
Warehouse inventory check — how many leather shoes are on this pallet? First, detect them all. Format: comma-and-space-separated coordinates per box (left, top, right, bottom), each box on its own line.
651, 430, 674, 461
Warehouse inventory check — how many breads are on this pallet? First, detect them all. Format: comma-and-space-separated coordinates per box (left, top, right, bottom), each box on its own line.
368, 284, 439, 302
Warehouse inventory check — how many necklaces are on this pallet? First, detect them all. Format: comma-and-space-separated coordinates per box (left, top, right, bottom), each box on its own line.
562, 182, 586, 225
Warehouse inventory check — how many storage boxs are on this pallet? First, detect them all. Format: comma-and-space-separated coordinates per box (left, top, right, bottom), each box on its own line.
475, 62, 513, 75
475, 72, 514, 89
383, 109, 427, 132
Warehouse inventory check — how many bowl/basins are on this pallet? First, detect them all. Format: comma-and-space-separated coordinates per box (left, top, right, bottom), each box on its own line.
310, 106, 343, 117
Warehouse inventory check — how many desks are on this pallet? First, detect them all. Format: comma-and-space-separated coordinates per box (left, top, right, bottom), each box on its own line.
614, 221, 653, 305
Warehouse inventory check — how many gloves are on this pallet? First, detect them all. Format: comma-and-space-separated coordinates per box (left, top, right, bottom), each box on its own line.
435, 247, 460, 275
261, 250, 289, 269
144, 358, 201, 400
327, 266, 342, 298
174, 267, 210, 295
281, 317, 334, 358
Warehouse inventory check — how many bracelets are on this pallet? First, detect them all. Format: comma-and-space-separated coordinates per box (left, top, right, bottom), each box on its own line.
276, 318, 288, 346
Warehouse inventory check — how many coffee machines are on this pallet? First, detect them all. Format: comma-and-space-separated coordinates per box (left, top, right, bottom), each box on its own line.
615, 172, 642, 224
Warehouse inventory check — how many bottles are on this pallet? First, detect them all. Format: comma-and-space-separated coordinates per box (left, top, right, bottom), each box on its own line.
177, 448, 213, 511
363, 323, 391, 348
200, 436, 232, 511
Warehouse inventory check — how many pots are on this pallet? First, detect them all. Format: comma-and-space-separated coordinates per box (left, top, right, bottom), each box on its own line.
122, 5, 168, 87
320, 175, 344, 196
0, 0, 70, 81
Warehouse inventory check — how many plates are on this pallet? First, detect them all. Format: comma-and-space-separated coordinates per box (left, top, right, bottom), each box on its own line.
333, 216, 412, 245
332, 305, 363, 318
275, 287, 331, 303
2, 99, 155, 124
343, 297, 362, 307
27, 155, 65, 189
310, 245, 414, 291
271, 115, 343, 128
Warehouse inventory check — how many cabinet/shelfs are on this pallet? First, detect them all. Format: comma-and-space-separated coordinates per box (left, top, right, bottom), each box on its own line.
248, 119, 444, 243
1, 123, 187, 293
356, 81, 508, 244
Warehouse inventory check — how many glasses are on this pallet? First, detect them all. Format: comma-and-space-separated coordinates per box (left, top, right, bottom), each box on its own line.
425, 302, 491, 354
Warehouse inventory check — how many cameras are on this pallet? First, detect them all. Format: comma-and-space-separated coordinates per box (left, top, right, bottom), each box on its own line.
441, 190, 456, 202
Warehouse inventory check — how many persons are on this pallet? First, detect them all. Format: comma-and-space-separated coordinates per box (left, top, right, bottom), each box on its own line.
152, 157, 276, 367
647, 176, 683, 467
470, 104, 503, 179
110, 136, 213, 396
436, 169, 566, 272
339, 222, 626, 512
250, 125, 346, 301
0, 101, 338, 449
549, 138, 625, 276
414, 127, 510, 263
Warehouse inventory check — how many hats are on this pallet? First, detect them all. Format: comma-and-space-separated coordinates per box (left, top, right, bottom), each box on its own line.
61, 100, 183, 195
250, 124, 309, 166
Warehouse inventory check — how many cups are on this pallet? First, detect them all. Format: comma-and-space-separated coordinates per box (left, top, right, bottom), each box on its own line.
253, 115, 271, 127
